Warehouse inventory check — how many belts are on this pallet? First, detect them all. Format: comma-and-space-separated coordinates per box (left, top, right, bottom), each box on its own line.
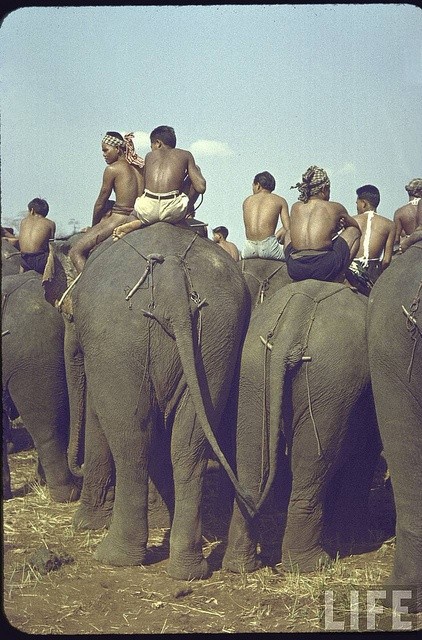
143, 192, 182, 200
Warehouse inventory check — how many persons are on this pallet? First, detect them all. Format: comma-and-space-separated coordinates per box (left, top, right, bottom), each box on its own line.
111, 125, 205, 241
284, 168, 360, 281
349, 184, 394, 290
67, 130, 145, 274
240, 172, 285, 262
394, 177, 422, 245
211, 224, 240, 262
15, 199, 58, 273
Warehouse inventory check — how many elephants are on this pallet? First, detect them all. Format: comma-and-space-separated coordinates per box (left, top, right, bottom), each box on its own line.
222, 279, 368, 575
368, 241, 422, 613
63, 222, 252, 579
236, 258, 292, 318
1, 241, 79, 504
